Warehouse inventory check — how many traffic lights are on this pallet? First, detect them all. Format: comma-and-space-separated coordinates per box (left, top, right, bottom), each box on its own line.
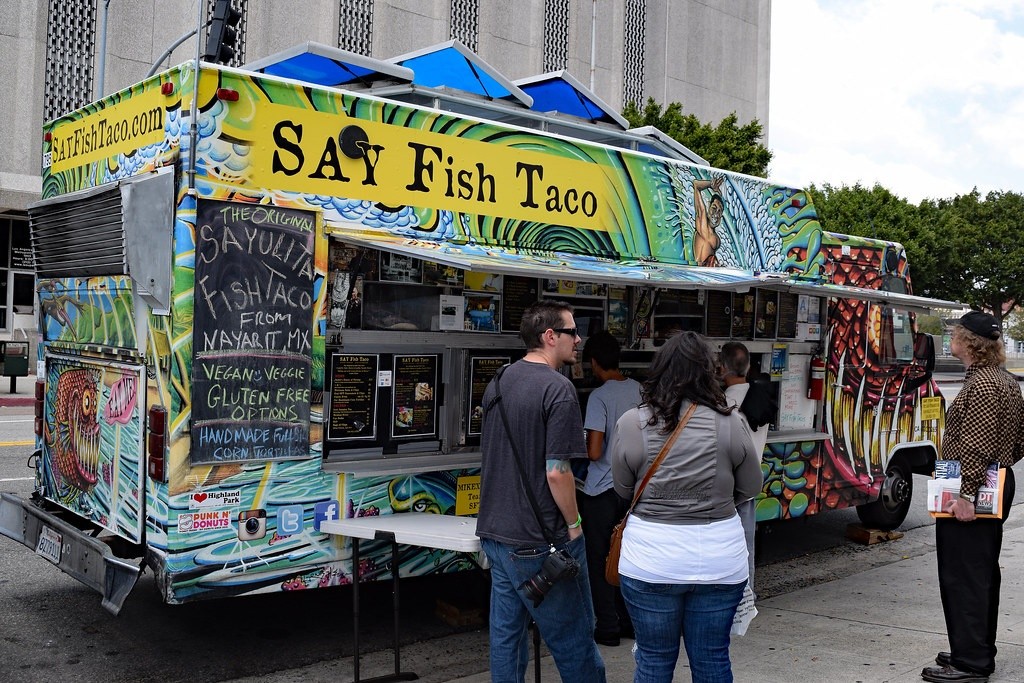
205, 0, 243, 64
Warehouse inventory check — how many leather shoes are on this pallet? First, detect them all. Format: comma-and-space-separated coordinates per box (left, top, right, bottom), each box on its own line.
921, 665, 989, 683
935, 652, 951, 667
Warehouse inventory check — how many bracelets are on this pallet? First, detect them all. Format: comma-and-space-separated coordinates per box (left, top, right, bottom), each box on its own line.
565, 512, 582, 528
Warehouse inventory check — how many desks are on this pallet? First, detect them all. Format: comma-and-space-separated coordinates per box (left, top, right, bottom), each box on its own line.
319, 512, 542, 683
323, 428, 831, 478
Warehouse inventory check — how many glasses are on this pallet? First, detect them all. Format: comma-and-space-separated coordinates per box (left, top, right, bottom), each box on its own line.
539, 328, 577, 338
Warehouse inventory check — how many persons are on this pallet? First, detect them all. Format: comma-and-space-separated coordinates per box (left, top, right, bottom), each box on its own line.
920, 311, 1024, 683
609, 332, 764, 682
717, 342, 775, 596
578, 331, 650, 646
475, 297, 607, 683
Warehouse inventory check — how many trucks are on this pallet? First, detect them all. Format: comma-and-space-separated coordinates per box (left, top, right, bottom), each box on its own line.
0, 55, 969, 634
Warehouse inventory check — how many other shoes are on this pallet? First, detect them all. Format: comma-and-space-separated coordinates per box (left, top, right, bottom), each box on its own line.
594, 626, 620, 646
618, 620, 636, 639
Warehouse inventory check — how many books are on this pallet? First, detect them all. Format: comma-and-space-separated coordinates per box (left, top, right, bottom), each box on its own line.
928, 460, 1007, 520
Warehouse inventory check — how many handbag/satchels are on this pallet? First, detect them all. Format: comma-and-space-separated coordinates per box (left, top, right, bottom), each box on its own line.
605, 524, 623, 586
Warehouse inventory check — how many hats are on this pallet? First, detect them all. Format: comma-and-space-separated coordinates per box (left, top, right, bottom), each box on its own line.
946, 311, 1002, 341
